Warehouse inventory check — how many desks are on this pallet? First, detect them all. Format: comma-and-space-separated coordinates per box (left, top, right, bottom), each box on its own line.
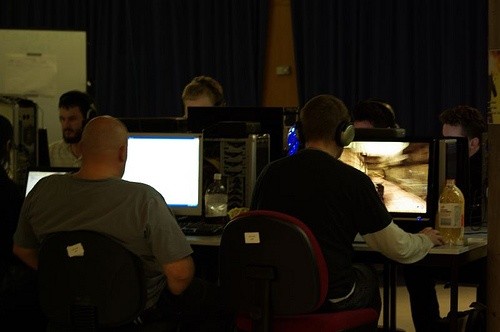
184, 226, 489, 331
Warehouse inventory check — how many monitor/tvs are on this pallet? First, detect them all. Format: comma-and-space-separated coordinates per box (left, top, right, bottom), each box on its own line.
38, 129, 50, 167
24, 167, 82, 202
337, 138, 436, 222
114, 117, 188, 132
121, 132, 203, 216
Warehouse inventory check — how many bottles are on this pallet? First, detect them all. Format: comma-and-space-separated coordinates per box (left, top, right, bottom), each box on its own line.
205, 173, 228, 217
438, 180, 465, 245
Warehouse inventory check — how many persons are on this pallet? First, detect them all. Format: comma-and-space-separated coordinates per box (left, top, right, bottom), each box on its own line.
181, 76, 225, 117
13, 115, 209, 332
47, 90, 99, 167
403, 105, 489, 332
250, 95, 446, 332
349, 100, 396, 129
0, 114, 25, 332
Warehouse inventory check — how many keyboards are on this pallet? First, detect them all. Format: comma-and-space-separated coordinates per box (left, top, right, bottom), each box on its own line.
178, 222, 225, 235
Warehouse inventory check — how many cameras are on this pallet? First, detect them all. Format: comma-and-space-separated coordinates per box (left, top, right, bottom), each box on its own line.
188, 106, 284, 159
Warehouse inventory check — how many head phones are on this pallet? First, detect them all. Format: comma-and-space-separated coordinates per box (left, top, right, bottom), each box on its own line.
86, 103, 99, 121
295, 119, 355, 146
198, 80, 225, 107
366, 101, 401, 128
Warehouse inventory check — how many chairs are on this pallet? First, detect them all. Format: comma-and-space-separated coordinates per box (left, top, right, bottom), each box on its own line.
25, 229, 148, 331
218, 209, 380, 332
